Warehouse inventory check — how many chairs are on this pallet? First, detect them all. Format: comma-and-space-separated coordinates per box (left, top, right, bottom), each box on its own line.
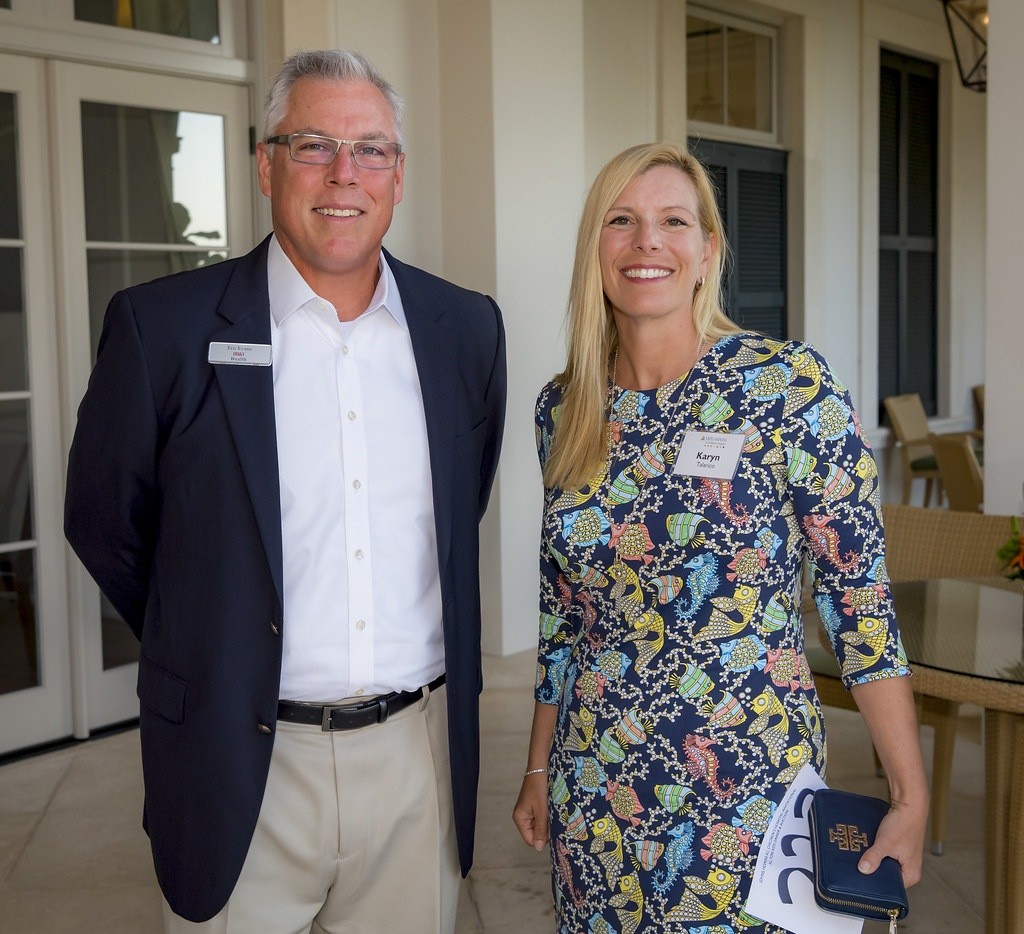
800, 504, 1024, 855
882, 386, 985, 513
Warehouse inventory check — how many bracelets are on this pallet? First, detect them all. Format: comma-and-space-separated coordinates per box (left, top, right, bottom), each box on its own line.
524, 769, 547, 778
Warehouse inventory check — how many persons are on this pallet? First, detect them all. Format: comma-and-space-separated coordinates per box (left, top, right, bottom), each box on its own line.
514, 144, 929, 934
62, 48, 506, 932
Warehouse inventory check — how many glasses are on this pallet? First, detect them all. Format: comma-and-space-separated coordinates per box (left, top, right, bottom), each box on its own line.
265, 131, 402, 170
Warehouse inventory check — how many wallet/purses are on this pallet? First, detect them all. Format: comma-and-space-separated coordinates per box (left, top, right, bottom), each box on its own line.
804, 786, 909, 934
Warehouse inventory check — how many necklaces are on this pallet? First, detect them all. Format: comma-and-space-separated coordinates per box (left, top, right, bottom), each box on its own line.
609, 314, 716, 625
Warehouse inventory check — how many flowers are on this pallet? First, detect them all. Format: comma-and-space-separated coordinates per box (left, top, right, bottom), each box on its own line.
995, 516, 1024, 581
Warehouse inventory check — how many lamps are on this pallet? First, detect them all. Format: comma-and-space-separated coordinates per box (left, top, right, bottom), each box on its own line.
690, 20, 741, 127
942, 0, 989, 92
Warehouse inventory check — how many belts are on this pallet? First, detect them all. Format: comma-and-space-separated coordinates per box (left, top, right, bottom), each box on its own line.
273, 668, 447, 733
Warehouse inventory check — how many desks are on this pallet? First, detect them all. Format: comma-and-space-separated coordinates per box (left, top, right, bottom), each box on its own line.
818, 576, 1024, 934
